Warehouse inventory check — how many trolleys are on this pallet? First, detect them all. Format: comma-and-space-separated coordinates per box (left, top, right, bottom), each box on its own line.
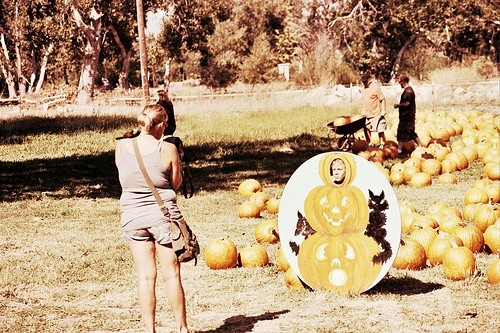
327, 114, 384, 153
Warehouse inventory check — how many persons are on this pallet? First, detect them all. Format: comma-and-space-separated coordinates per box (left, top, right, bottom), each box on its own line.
330, 158, 346, 184
114, 104, 195, 333
394, 74, 421, 155
360, 74, 387, 144
156, 90, 176, 136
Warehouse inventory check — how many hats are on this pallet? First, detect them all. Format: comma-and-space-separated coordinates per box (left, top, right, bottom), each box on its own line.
360, 74, 371, 83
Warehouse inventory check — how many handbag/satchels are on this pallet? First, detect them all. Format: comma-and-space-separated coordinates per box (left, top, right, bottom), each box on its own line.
168, 214, 200, 266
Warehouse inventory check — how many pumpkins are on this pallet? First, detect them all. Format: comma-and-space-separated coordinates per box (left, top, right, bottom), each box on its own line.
332, 107, 500, 287
203, 178, 304, 290
